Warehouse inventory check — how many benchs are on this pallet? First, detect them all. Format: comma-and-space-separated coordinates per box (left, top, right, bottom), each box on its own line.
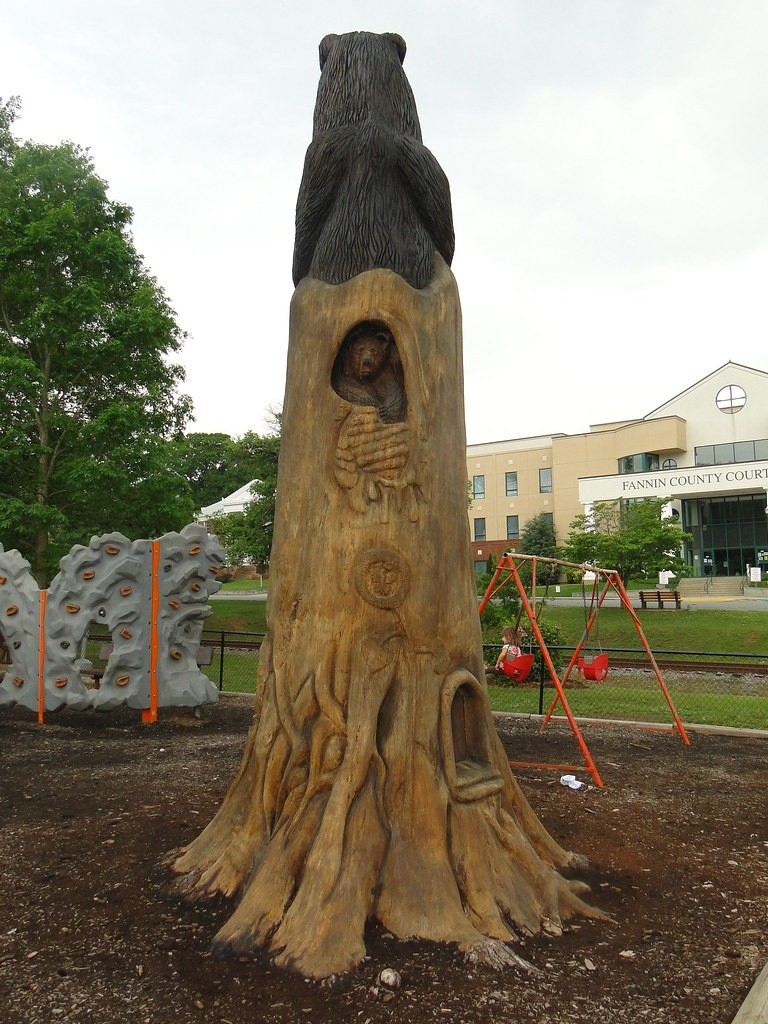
638, 591, 684, 609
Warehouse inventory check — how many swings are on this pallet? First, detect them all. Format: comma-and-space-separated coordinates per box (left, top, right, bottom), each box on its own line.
580, 563, 610, 682
496, 559, 558, 683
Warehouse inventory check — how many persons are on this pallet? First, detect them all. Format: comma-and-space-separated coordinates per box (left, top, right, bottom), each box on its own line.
482, 625, 523, 675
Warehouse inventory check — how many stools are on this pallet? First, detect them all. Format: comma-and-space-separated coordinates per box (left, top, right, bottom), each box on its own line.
77, 664, 107, 689
